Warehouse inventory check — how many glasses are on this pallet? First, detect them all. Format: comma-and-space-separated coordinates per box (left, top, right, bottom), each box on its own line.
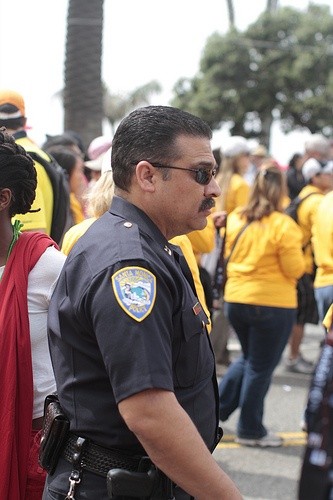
129, 160, 215, 185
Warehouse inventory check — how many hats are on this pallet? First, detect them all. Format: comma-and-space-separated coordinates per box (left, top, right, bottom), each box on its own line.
218, 136, 260, 158
301, 156, 333, 180
0, 91, 25, 120
88, 135, 113, 158
84, 152, 105, 171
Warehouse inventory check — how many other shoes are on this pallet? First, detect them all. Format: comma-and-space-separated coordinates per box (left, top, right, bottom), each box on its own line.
286, 356, 315, 374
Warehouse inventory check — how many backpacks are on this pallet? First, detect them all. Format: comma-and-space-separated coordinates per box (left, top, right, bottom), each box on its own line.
284, 191, 319, 253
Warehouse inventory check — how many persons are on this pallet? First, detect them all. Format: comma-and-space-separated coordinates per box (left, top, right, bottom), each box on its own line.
187, 138, 333, 500
0, 90, 212, 333
43, 105, 244, 500
0, 126, 67, 500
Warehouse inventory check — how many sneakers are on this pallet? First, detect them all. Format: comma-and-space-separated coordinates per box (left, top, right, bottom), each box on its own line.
234, 429, 284, 447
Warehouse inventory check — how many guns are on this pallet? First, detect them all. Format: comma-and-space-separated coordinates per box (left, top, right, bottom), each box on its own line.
39, 395, 70, 476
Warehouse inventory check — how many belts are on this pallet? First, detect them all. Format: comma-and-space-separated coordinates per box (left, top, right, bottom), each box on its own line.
60, 437, 129, 478
